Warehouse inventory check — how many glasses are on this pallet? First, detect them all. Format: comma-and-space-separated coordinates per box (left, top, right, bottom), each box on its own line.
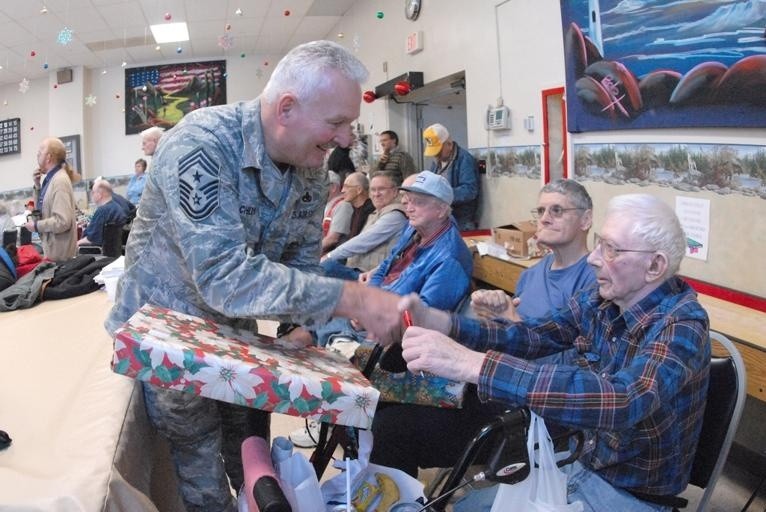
342, 185, 396, 191
593, 231, 657, 262
530, 204, 585, 219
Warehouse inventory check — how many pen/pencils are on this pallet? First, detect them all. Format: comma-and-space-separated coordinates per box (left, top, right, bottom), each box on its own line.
401, 310, 424, 378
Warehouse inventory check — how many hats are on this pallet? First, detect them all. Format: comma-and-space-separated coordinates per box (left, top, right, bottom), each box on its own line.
422, 123, 449, 157
396, 170, 455, 207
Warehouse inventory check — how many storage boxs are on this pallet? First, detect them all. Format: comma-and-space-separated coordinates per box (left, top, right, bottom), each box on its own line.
496, 219, 544, 258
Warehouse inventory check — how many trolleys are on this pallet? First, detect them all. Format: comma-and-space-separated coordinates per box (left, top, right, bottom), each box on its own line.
224, 314, 532, 512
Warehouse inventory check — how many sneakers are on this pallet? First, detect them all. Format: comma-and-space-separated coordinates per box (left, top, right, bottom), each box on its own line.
290, 419, 333, 448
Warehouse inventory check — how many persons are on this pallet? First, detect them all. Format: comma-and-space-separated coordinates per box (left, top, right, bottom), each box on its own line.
0, 201, 17, 246
367, 192, 712, 512
11, 199, 32, 226
89, 176, 135, 217
76, 179, 133, 256
127, 158, 145, 204
370, 177, 598, 473
376, 130, 416, 180
103, 40, 371, 512
22, 136, 79, 261
424, 123, 479, 227
139, 122, 162, 155
278, 170, 473, 349
325, 145, 355, 174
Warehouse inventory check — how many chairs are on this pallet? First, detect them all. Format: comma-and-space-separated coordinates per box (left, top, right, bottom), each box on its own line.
3, 207, 137, 258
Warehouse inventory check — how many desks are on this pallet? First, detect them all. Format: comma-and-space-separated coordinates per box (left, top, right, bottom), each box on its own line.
0, 286, 178, 511
460, 228, 766, 402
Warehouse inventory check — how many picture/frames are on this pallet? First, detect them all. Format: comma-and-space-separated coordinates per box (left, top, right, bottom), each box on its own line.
57, 134, 81, 176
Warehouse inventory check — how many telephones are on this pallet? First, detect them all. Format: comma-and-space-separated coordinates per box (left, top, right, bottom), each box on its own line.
482, 104, 511, 130
